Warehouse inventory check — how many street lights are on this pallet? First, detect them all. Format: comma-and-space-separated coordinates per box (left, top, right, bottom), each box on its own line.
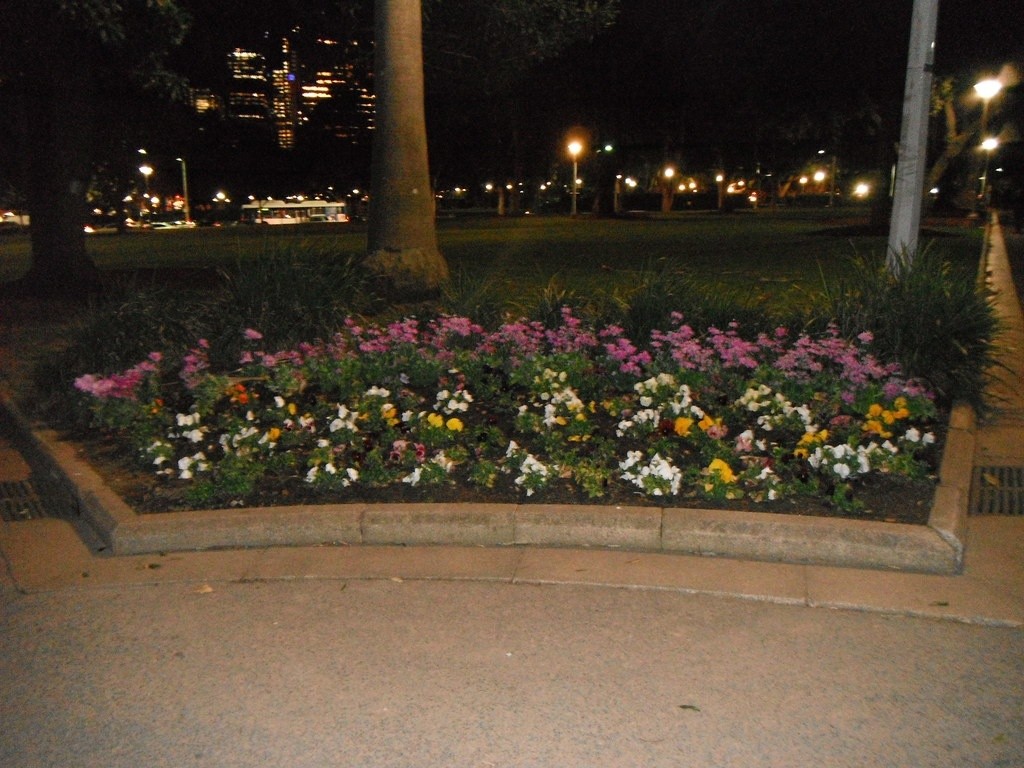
248, 194, 274, 223
971, 77, 1004, 170
567, 140, 581, 219
352, 188, 360, 206
715, 172, 723, 209
138, 163, 154, 228
977, 137, 1000, 211
175, 156, 191, 224
